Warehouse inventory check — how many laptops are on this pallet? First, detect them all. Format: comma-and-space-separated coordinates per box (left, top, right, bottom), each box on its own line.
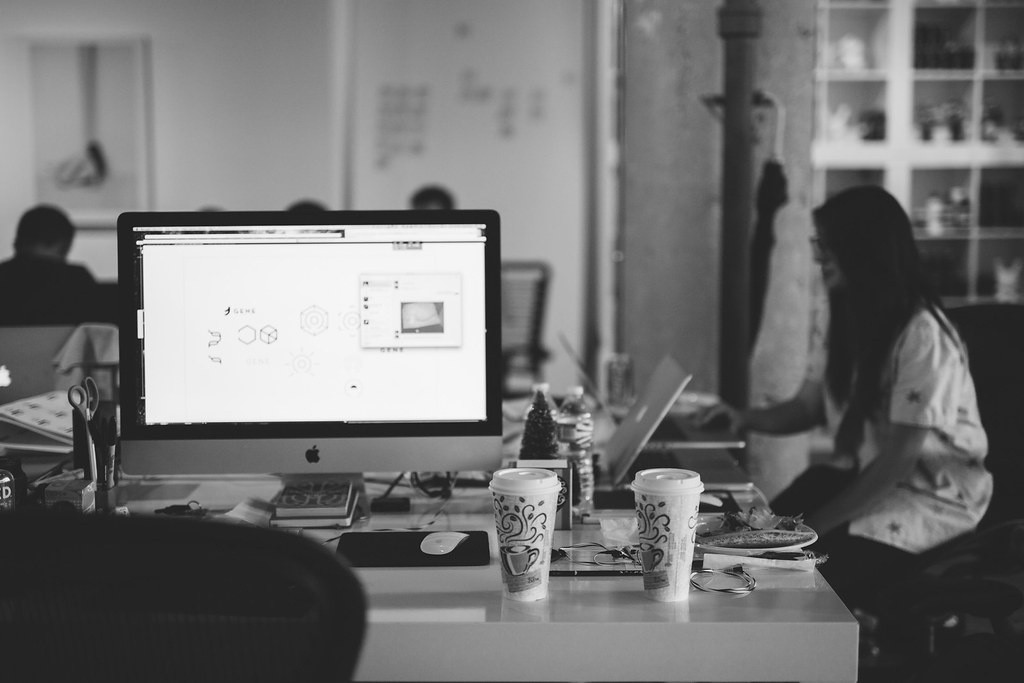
603, 355, 753, 492
557, 333, 747, 450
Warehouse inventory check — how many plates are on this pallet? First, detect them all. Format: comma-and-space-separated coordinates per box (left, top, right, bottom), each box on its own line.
695, 512, 818, 556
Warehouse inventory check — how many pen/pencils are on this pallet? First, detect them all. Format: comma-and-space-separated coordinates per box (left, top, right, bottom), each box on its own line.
87, 416, 121, 491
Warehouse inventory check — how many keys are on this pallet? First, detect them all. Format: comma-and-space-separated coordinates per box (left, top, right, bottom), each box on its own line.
154, 501, 208, 516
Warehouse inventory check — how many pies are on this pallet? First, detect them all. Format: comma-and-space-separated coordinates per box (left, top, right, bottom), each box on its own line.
696, 529, 815, 546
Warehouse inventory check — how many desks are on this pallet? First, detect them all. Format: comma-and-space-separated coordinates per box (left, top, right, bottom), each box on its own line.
0, 399, 859, 683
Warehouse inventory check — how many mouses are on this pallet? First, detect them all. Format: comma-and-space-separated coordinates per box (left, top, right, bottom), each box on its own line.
420, 532, 470, 555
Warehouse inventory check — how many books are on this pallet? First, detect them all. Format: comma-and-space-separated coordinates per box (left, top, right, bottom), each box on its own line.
269, 479, 359, 529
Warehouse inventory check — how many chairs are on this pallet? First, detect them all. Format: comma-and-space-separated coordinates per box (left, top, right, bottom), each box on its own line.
857, 305, 1024, 683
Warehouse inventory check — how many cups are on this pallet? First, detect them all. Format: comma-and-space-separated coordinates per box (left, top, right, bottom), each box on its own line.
488, 468, 562, 602
630, 468, 705, 601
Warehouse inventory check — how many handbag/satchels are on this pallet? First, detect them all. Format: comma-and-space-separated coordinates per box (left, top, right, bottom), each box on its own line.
765, 462, 858, 553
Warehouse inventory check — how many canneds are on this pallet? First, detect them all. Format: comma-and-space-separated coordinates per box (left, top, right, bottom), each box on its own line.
603, 352, 634, 409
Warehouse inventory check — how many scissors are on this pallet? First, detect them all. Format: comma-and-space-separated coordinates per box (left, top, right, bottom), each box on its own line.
67, 376, 101, 433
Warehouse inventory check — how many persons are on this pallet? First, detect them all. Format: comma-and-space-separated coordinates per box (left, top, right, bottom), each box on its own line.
693, 189, 993, 669
0, 205, 106, 325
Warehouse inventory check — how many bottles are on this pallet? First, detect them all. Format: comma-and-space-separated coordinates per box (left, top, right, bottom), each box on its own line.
557, 386, 594, 515
608, 352, 631, 407
523, 383, 558, 444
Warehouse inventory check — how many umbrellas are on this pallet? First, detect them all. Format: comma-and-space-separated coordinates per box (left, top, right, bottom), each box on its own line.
741, 88, 790, 357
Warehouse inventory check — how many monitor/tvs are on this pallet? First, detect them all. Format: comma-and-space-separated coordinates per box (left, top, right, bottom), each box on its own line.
116, 210, 502, 528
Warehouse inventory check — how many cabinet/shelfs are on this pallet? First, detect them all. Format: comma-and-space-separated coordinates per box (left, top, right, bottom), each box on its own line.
815, 0, 1024, 305
